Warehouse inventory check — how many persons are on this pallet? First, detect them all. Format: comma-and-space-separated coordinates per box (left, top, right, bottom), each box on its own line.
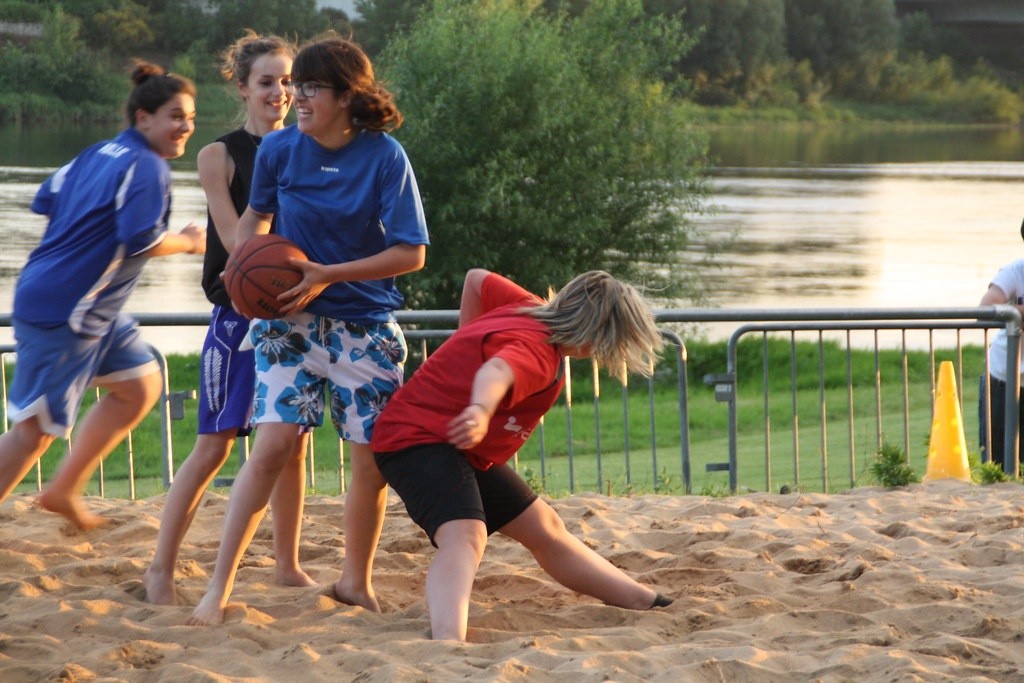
979, 218, 1024, 473
371, 269, 674, 642
144, 32, 430, 629
0, 61, 196, 532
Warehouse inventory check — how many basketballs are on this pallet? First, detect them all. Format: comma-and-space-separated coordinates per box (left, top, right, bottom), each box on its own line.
224, 233, 310, 321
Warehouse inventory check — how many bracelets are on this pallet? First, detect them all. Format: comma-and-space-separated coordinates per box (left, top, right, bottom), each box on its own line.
469, 403, 491, 417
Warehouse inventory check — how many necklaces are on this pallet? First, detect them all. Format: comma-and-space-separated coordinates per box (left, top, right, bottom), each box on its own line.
245, 127, 259, 148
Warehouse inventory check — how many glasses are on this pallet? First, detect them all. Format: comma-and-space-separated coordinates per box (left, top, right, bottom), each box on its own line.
288, 81, 337, 98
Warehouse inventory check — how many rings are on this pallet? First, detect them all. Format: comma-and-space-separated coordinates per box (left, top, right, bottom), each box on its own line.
465, 420, 476, 426
